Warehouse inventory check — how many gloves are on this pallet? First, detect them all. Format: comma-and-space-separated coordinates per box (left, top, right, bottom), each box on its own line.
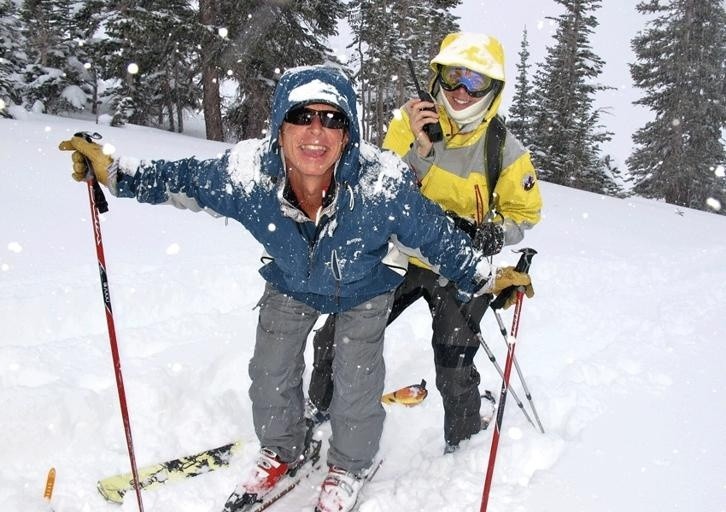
476, 265, 535, 310
58, 132, 118, 197
445, 209, 505, 257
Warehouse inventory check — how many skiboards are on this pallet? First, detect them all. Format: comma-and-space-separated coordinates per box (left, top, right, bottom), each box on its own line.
98, 380, 428, 507
221, 438, 324, 512
98, 379, 495, 512
314, 455, 384, 512
476, 389, 496, 431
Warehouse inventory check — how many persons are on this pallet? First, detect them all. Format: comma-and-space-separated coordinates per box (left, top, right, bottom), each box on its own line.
59, 64, 535, 512
303, 31, 541, 455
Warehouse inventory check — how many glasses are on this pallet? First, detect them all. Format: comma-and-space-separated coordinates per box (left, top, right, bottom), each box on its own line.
436, 65, 495, 96
283, 105, 349, 130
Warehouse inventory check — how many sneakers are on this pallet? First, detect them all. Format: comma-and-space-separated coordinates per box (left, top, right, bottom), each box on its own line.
303, 398, 332, 424
315, 465, 365, 512
243, 448, 301, 500
